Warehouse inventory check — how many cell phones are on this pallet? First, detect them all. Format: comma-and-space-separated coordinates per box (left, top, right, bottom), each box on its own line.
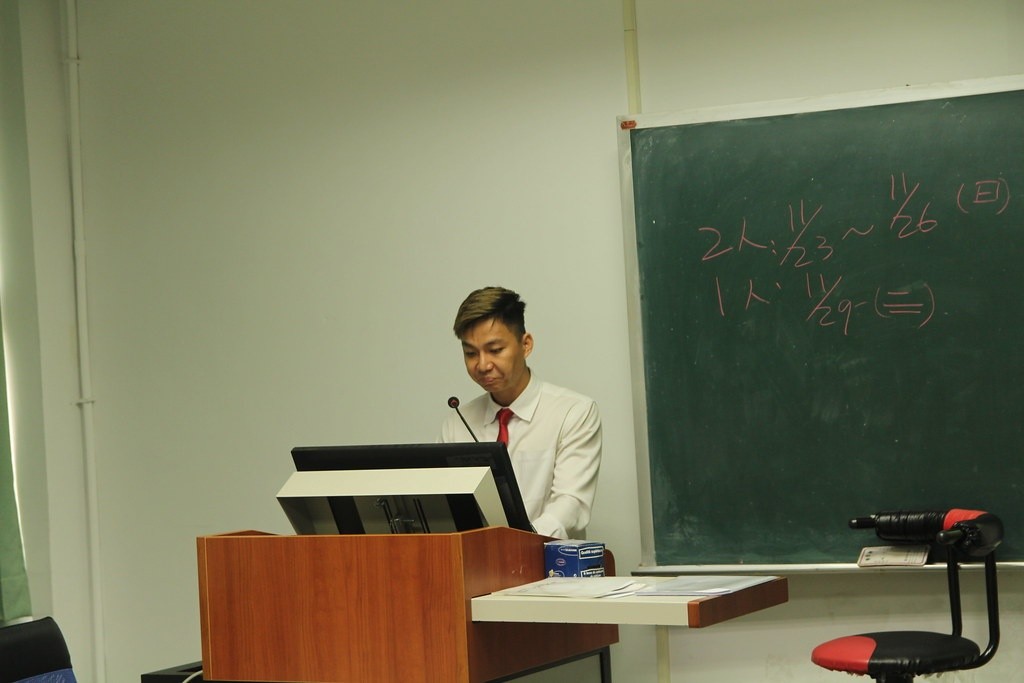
857, 545, 930, 567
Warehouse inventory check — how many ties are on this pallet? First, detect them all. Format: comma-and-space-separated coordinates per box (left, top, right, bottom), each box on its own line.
496, 407, 514, 447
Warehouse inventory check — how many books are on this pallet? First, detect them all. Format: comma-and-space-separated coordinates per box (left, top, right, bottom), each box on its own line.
636, 576, 779, 596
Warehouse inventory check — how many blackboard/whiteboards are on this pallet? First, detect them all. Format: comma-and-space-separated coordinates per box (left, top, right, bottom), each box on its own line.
613, 77, 1024, 577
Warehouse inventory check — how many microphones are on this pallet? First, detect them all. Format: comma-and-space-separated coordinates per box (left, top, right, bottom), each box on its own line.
448, 397, 479, 442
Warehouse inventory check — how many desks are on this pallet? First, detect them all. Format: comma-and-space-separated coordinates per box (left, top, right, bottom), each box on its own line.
471, 573, 791, 682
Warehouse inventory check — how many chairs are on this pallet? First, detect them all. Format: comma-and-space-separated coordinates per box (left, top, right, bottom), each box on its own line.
812, 509, 1005, 683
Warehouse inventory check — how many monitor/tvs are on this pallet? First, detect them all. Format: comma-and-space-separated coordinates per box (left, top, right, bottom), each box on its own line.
292, 442, 532, 532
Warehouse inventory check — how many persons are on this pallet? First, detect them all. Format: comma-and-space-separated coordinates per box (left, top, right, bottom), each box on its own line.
437, 287, 602, 541
